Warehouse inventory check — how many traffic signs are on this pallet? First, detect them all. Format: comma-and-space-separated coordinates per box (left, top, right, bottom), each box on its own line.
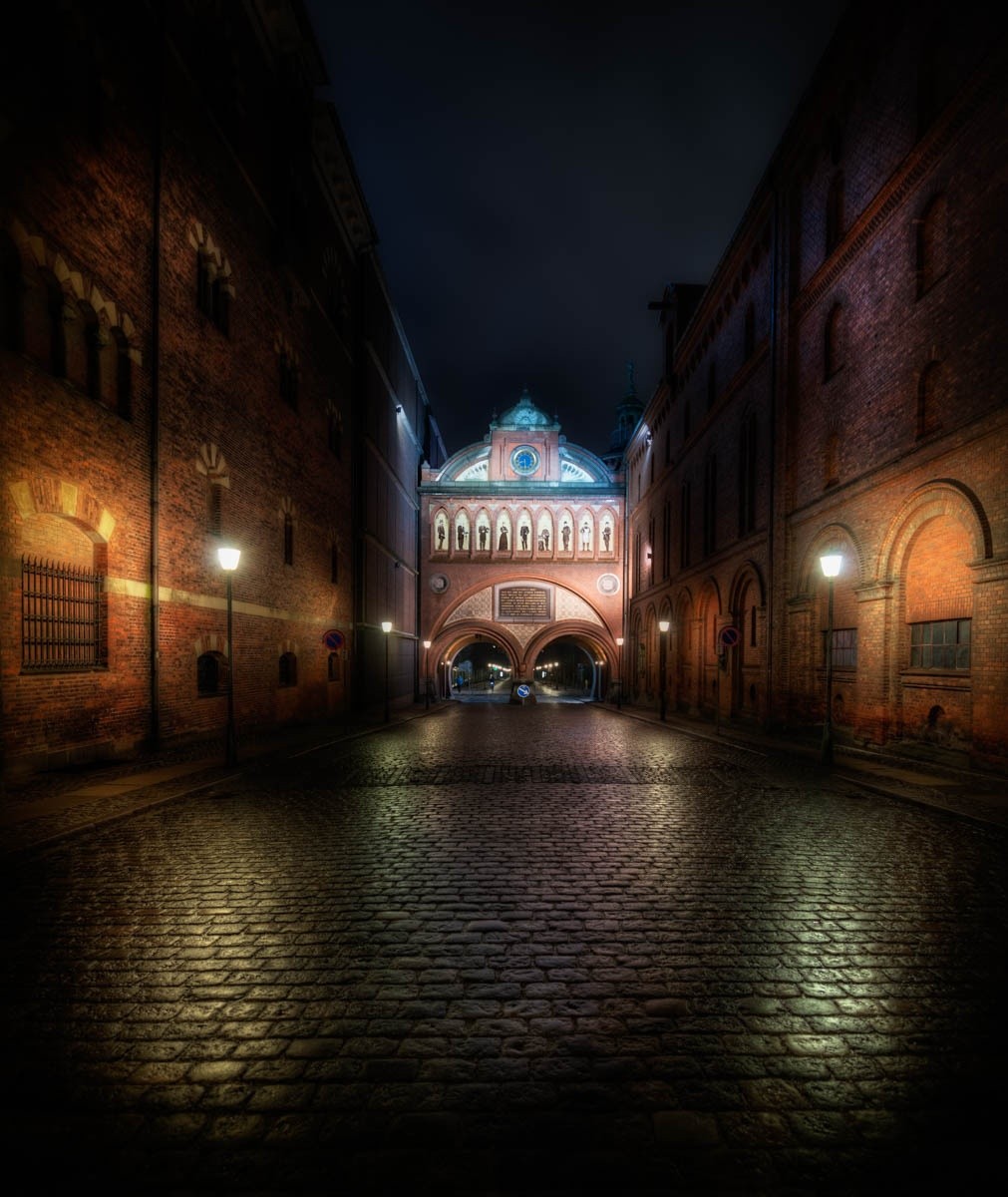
518, 685, 530, 699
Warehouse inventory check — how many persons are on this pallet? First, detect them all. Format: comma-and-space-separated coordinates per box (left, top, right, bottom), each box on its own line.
456, 675, 464, 693
490, 677, 495, 691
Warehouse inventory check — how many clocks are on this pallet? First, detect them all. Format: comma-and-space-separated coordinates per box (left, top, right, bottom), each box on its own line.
510, 445, 541, 475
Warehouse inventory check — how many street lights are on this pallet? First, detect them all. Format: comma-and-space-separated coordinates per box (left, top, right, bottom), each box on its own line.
616, 636, 625, 710
423, 641, 431, 709
487, 664, 511, 682
818, 554, 843, 754
658, 620, 671, 720
218, 547, 241, 766
381, 621, 392, 720
535, 662, 559, 690
